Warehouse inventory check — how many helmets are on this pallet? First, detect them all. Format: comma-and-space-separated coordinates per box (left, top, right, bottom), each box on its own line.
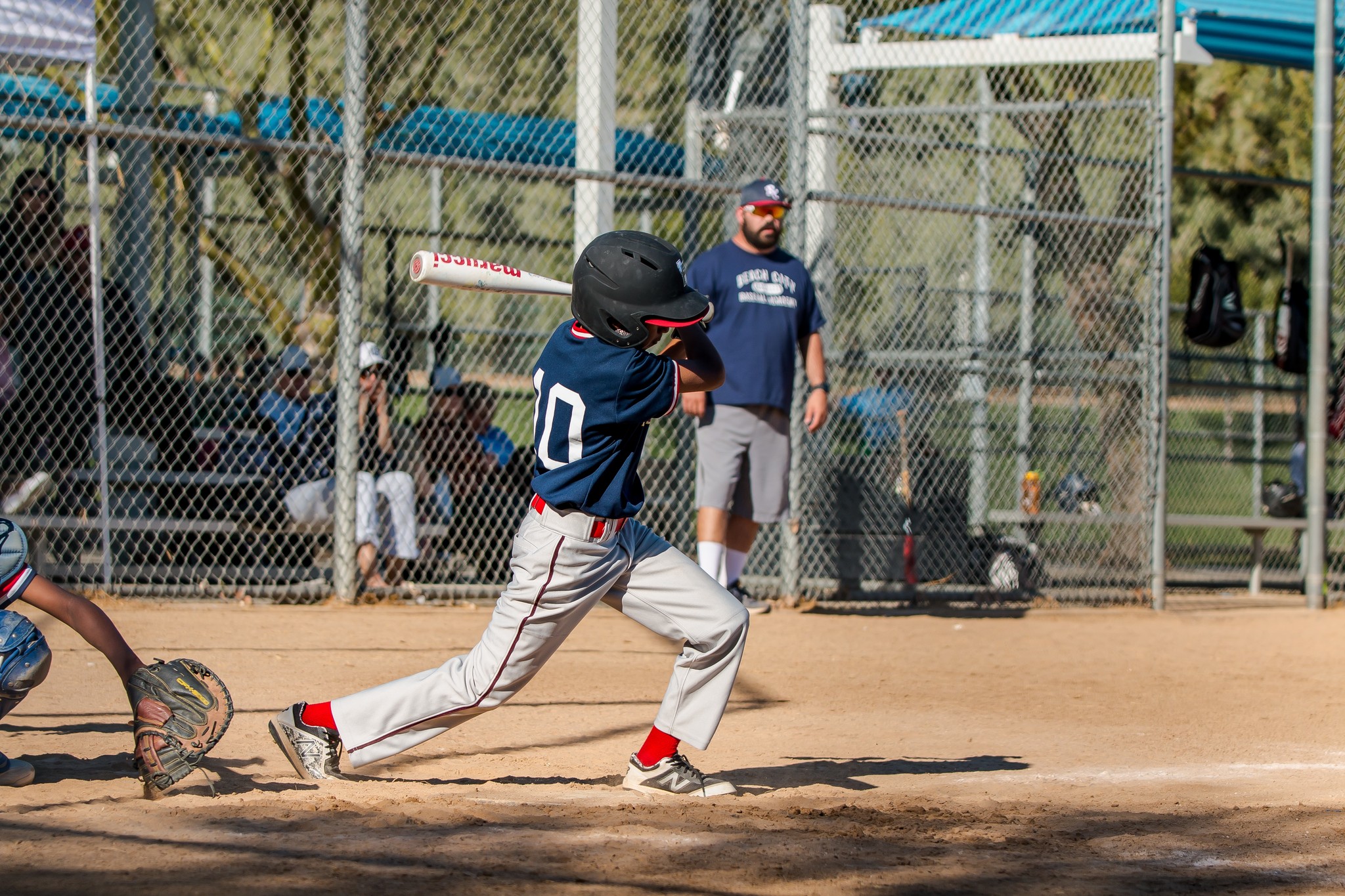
571, 230, 709, 348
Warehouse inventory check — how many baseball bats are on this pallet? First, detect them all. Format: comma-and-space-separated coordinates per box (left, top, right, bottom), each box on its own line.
896, 409, 917, 607
408, 249, 573, 296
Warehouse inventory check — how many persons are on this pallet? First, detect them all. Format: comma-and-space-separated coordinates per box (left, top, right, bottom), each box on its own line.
268, 231, 749, 798
0, 518, 233, 790
1286, 422, 1308, 505
681, 179, 827, 614
0, 169, 272, 585
256, 341, 537, 587
836, 350, 935, 501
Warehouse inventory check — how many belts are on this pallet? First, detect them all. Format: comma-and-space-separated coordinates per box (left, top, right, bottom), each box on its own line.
532, 494, 629, 538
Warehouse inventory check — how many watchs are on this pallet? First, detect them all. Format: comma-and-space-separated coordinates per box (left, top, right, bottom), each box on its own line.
810, 382, 829, 393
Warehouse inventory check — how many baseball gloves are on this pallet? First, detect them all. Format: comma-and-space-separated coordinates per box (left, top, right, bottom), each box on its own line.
126, 658, 235, 796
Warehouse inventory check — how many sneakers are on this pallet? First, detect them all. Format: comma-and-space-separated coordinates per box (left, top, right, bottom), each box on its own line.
0, 752, 35, 787
268, 701, 351, 781
622, 750, 738, 798
727, 578, 771, 613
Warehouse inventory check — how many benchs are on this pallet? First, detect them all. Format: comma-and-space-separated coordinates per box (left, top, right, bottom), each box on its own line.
987, 509, 1345, 602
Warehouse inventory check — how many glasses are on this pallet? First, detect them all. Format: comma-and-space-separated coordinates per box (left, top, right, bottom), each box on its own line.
360, 368, 386, 379
285, 368, 312, 378
744, 205, 788, 220
437, 386, 466, 397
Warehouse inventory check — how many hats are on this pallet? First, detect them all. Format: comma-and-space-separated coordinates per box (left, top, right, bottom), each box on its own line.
644, 304, 710, 327
360, 341, 394, 372
740, 178, 793, 209
277, 344, 312, 368
429, 366, 462, 391
